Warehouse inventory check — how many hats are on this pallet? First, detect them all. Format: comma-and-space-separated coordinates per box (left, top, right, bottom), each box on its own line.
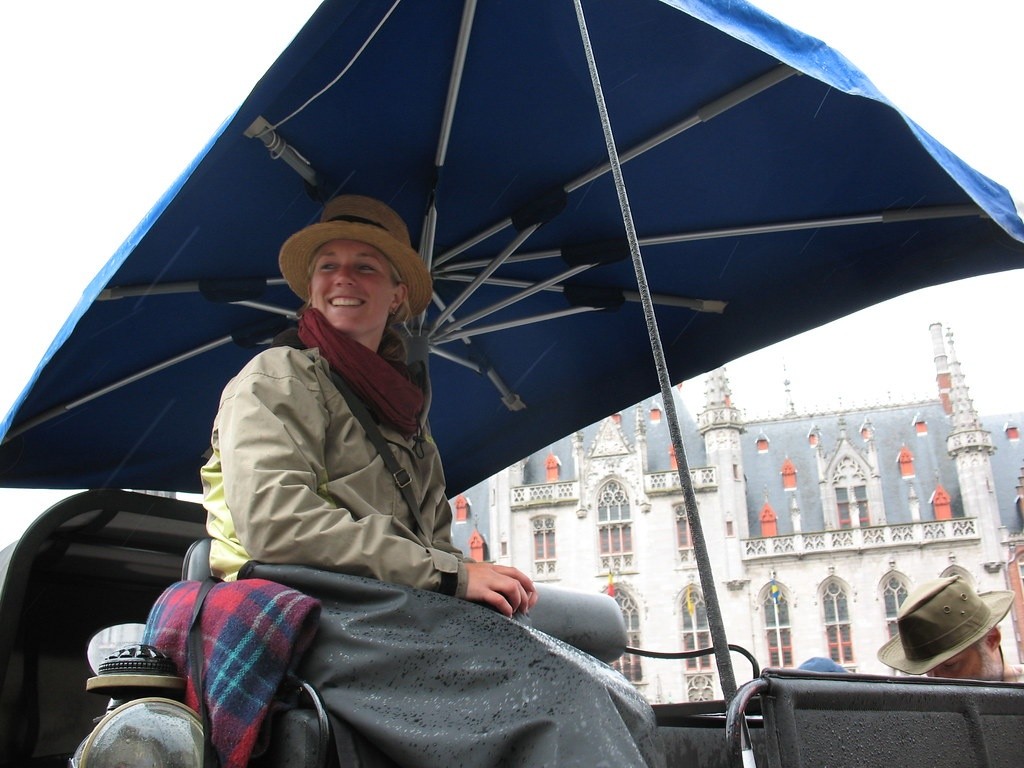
278, 195, 432, 324
876, 576, 1015, 675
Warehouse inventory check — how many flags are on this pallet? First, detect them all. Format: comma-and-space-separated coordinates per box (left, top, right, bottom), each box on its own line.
769, 578, 782, 606
687, 585, 696, 614
608, 573, 615, 597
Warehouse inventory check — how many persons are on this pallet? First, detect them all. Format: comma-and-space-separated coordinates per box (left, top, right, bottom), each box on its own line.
198, 196, 659, 768
876, 574, 1024, 684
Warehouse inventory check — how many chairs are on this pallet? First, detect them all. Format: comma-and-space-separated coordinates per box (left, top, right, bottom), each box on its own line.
179, 534, 332, 768
